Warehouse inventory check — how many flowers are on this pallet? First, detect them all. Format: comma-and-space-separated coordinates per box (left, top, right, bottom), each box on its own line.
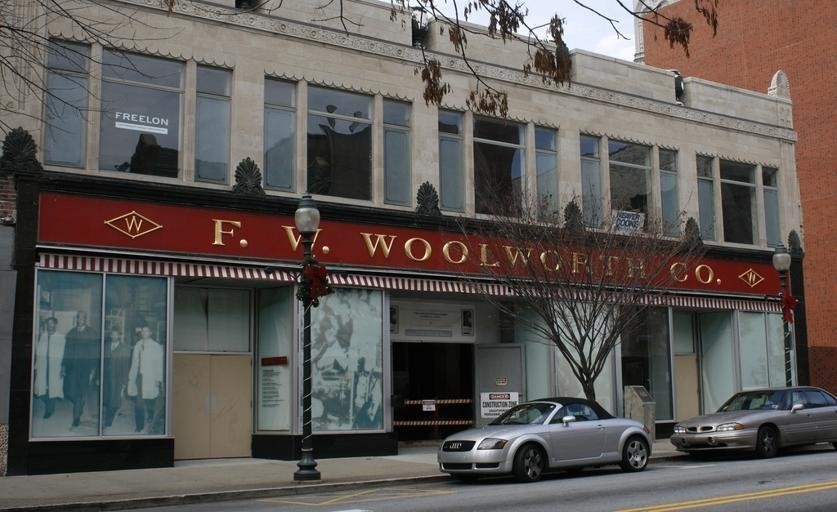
295, 262, 336, 310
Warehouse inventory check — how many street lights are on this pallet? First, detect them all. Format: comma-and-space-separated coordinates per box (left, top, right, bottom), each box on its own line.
293, 190, 321, 481
772, 243, 791, 387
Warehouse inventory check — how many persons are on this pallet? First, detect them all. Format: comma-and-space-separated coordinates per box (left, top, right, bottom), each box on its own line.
34, 310, 164, 432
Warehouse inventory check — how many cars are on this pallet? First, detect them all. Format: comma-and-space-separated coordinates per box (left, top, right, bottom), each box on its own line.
670, 386, 837, 459
437, 397, 653, 482
